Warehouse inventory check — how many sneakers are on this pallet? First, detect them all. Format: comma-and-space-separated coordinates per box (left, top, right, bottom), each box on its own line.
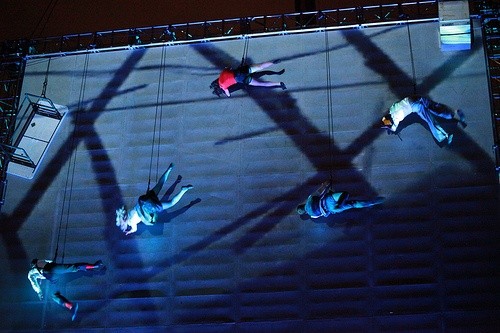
181, 184, 194, 191
454, 109, 467, 129
280, 81, 287, 90
273, 58, 282, 65
447, 133, 454, 146
170, 161, 175, 169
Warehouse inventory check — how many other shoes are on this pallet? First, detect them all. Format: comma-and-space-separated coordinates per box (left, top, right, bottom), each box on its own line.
68, 302, 81, 323
95, 260, 106, 271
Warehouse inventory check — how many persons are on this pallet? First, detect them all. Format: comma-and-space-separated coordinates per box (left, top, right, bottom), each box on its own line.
115, 162, 195, 235
209, 58, 285, 97
297, 176, 386, 219
379, 95, 465, 144
28, 258, 104, 321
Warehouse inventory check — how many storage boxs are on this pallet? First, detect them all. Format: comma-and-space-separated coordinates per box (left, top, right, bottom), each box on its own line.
438, 0, 471, 34
